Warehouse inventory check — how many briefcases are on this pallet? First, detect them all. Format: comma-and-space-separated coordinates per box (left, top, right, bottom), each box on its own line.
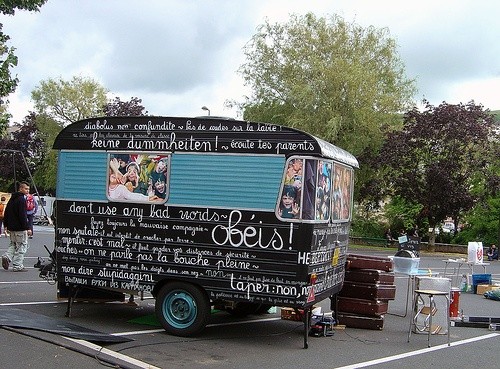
331, 253, 396, 330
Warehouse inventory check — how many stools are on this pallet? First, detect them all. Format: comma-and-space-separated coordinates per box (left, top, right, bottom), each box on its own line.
408, 289, 450, 348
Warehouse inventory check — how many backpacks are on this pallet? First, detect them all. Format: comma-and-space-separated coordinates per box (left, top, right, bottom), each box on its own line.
0, 203, 7, 217
25, 194, 34, 211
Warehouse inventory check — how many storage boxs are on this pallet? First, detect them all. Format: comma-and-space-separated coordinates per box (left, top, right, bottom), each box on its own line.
281, 305, 322, 322
473, 274, 492, 294
330, 255, 420, 332
477, 285, 500, 295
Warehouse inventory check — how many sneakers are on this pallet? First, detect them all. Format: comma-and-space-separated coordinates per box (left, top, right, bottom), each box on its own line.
13, 267, 28, 272
2, 255, 9, 270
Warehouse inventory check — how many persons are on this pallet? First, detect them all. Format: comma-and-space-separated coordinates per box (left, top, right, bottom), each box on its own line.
384, 229, 393, 248
400, 229, 407, 236
2, 182, 32, 272
486, 244, 498, 261
412, 224, 421, 237
0, 197, 7, 237
24, 195, 38, 239
109, 155, 167, 202
279, 159, 351, 219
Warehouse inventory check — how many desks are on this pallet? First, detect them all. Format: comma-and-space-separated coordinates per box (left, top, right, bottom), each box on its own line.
387, 268, 439, 318
442, 260, 490, 287
412, 276, 452, 335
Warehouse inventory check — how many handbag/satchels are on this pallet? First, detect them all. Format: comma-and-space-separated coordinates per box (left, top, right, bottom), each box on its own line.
468, 242, 483, 265
